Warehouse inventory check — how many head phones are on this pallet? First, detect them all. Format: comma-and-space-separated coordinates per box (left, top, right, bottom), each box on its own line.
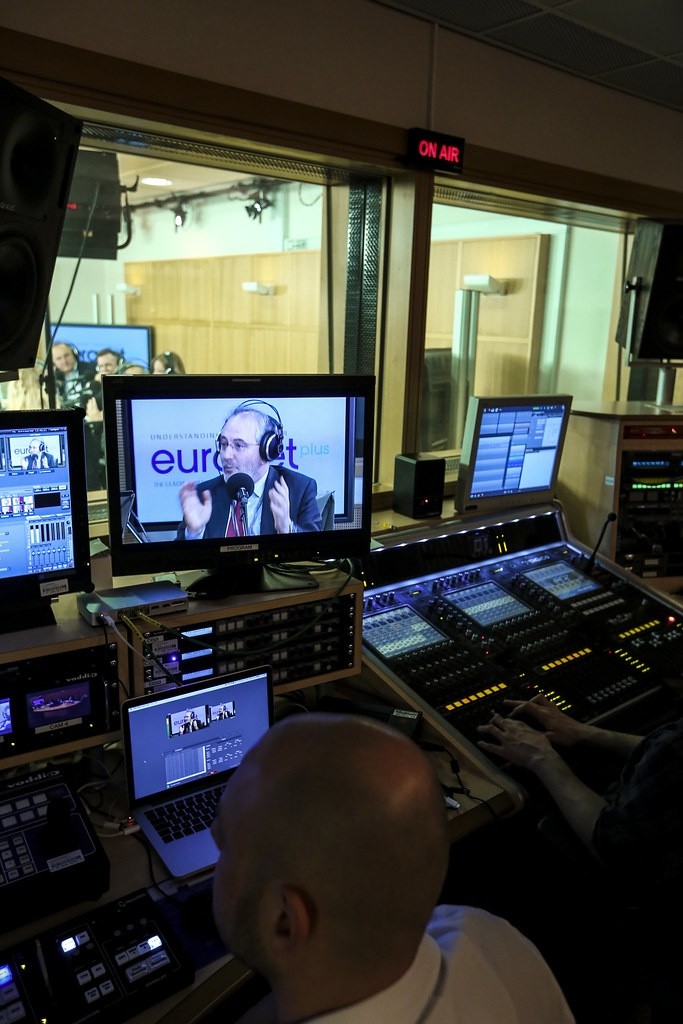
31, 438, 44, 451
213, 400, 283, 462
161, 351, 174, 374
95, 353, 124, 373
67, 343, 79, 357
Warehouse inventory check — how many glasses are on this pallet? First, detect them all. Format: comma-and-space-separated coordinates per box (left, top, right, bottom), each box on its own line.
95, 362, 119, 371
220, 441, 260, 451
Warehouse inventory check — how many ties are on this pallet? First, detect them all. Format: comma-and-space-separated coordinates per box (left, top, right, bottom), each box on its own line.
33, 460, 37, 469
226, 502, 244, 537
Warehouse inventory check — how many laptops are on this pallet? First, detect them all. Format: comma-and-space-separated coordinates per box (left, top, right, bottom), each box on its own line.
120, 664, 276, 880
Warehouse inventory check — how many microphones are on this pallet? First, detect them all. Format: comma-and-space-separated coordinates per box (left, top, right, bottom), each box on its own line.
31, 454, 38, 462
226, 472, 255, 504
577, 512, 617, 576
54, 372, 101, 393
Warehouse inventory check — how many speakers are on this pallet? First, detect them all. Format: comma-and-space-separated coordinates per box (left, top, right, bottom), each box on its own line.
613, 216, 683, 360
392, 452, 446, 518
0, 79, 82, 373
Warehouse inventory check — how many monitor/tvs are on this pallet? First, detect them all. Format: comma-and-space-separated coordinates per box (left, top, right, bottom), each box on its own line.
121, 398, 355, 532
453, 393, 573, 514
0, 408, 88, 635
99, 373, 377, 598
49, 324, 154, 374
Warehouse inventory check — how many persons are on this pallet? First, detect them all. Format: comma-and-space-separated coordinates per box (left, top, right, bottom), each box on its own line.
210, 714, 575, 1024
175, 408, 322, 540
178, 711, 204, 735
21, 439, 54, 470
476, 694, 683, 1024
216, 705, 233, 720
43, 343, 186, 490
